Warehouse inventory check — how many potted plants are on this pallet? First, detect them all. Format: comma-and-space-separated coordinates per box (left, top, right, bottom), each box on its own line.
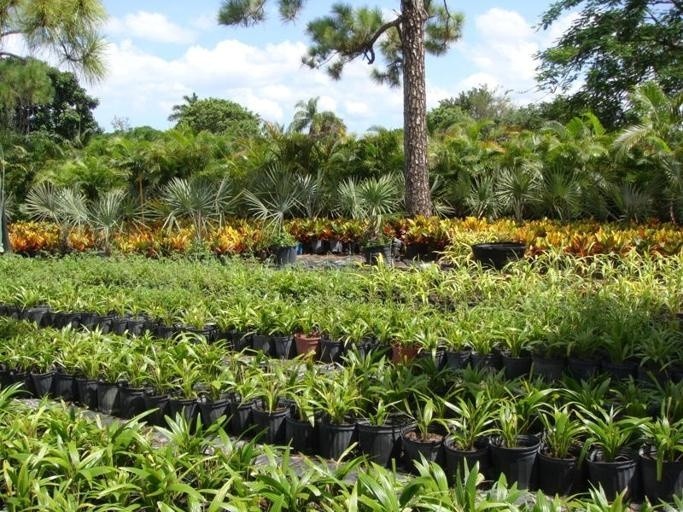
1, 213, 682, 511
